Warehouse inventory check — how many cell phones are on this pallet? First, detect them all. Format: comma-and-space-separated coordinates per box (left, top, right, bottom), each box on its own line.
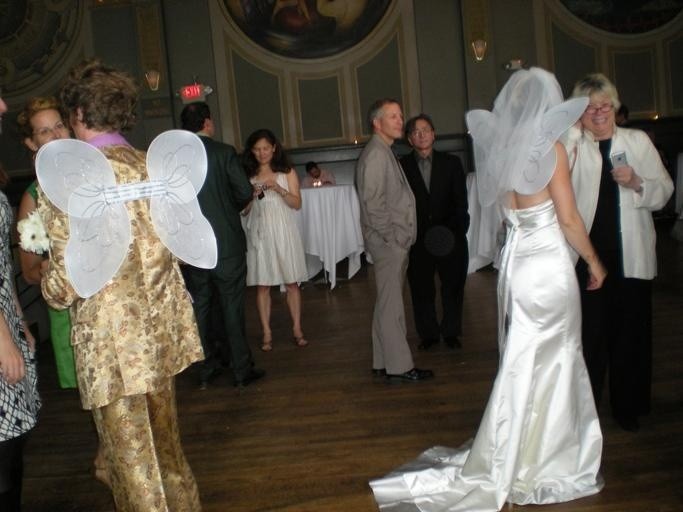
611, 151, 627, 170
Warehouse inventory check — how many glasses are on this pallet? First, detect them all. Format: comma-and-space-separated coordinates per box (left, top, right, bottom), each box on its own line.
582, 102, 615, 114
28, 120, 70, 137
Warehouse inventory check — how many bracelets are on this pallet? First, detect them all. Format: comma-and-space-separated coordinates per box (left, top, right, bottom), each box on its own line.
279, 188, 288, 198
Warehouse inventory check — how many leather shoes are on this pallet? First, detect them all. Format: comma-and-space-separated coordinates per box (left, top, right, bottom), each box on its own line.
414, 336, 463, 349
197, 367, 266, 391
262, 336, 273, 352
372, 365, 435, 384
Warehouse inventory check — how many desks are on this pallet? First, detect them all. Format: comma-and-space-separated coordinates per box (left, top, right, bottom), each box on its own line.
277, 185, 374, 293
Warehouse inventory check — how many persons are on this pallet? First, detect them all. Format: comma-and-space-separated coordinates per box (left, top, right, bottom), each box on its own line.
566, 73, 675, 431
369, 67, 608, 512
17, 96, 111, 484
354, 98, 433, 382
399, 114, 469, 349
16, 63, 219, 512
0, 98, 42, 512
178, 102, 264, 389
615, 104, 683, 256
300, 162, 335, 189
238, 129, 308, 351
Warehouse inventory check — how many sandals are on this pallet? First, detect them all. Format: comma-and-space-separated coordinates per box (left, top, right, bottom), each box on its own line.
293, 334, 309, 349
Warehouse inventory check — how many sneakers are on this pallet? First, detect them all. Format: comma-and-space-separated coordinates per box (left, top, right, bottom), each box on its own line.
611, 411, 640, 435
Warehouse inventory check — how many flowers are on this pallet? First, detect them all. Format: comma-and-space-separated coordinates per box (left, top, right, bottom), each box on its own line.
15, 207, 49, 255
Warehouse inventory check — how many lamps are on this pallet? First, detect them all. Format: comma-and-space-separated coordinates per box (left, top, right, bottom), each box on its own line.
143, 63, 161, 91
470, 31, 489, 64
